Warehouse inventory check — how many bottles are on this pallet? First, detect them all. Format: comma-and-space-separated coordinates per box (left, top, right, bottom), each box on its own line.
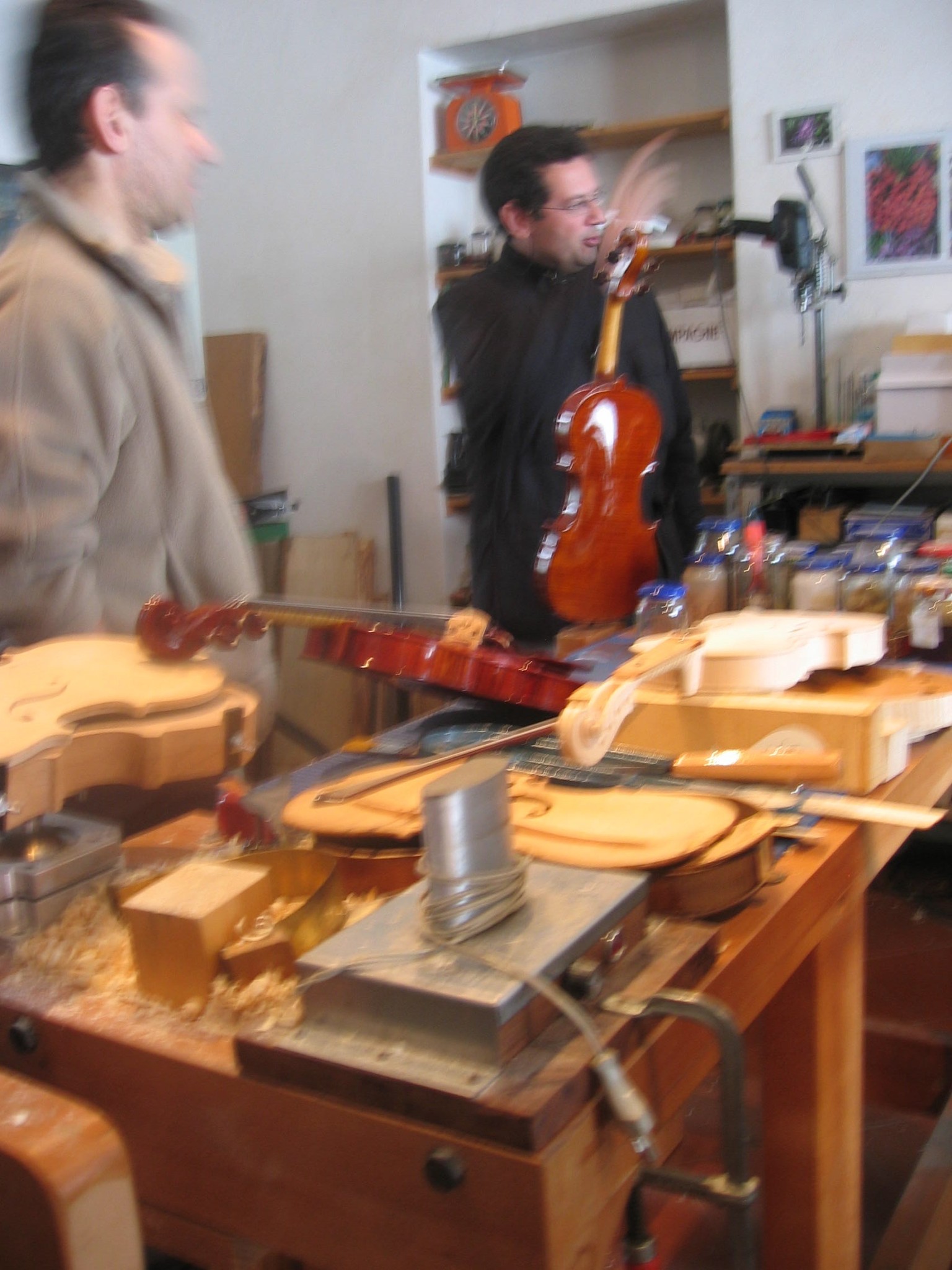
633, 515, 952, 659
438, 226, 509, 267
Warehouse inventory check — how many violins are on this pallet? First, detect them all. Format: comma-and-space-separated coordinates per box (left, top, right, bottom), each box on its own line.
527, 224, 670, 623
137, 591, 592, 715
556, 608, 895, 769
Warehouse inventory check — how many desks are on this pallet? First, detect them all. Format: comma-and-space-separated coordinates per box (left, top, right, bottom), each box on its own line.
0, 615, 952, 1270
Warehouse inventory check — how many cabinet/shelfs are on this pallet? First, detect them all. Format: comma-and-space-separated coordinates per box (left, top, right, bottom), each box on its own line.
429, 112, 737, 511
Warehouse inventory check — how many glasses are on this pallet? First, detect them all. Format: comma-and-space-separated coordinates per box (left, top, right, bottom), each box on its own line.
539, 192, 605, 215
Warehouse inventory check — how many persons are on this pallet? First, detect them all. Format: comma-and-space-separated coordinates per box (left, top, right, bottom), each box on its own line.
0, 1, 280, 744
434, 126, 702, 660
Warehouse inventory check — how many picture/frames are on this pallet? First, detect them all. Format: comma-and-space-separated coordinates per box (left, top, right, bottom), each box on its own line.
768, 105, 840, 164
841, 127, 952, 283
